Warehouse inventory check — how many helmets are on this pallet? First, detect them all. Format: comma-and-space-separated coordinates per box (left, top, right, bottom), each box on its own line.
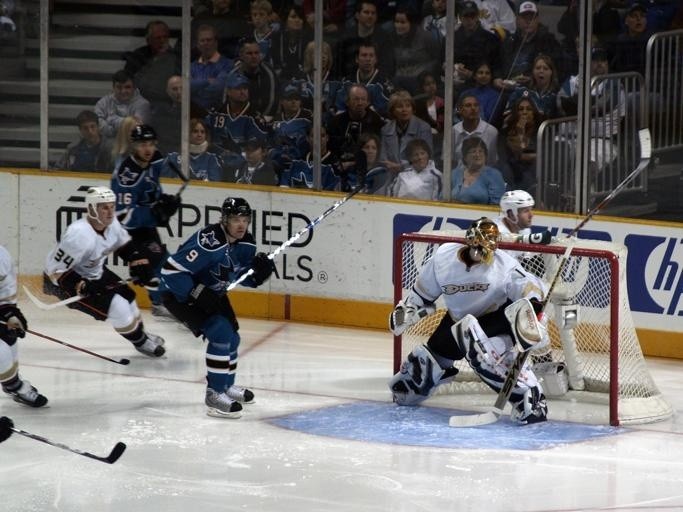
85, 186, 116, 219
221, 197, 252, 218
500, 190, 536, 225
465, 217, 502, 265
129, 124, 158, 145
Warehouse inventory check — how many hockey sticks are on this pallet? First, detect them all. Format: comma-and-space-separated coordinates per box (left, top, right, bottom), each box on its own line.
167, 159, 189, 196
0, 319, 130, 366
10, 426, 126, 464
218, 151, 366, 297
565, 129, 652, 237
448, 235, 577, 427
22, 275, 140, 310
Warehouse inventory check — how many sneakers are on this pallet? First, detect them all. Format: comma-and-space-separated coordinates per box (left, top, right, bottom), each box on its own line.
3, 305, 254, 412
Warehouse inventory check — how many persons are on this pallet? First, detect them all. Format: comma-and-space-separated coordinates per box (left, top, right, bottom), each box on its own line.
41, 185, 168, 358
52, 1, 682, 208
107, 122, 179, 320
387, 217, 550, 426
1, 247, 50, 408
491, 189, 554, 365
156, 195, 274, 414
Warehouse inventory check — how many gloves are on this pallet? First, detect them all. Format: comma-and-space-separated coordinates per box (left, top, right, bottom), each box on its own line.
186, 283, 226, 316
58, 268, 111, 300
245, 254, 276, 290
118, 239, 156, 288
149, 193, 183, 228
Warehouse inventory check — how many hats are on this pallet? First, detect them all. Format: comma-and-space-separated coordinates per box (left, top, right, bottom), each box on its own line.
518, 1, 537, 13
227, 72, 251, 89
